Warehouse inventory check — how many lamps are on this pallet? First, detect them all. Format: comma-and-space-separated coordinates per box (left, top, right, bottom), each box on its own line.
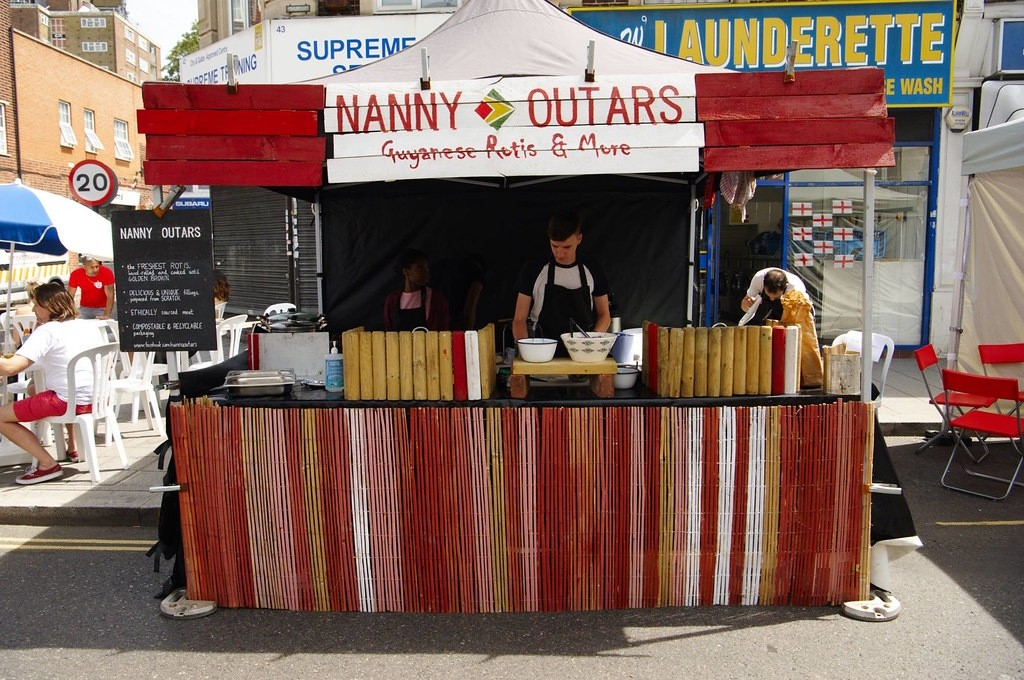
286, 4, 310, 15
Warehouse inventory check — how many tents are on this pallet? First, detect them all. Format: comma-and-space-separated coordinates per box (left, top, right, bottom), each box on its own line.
769, 168, 923, 258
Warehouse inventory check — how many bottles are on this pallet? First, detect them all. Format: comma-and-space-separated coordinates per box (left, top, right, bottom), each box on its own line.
22, 329, 30, 345
325, 341, 344, 392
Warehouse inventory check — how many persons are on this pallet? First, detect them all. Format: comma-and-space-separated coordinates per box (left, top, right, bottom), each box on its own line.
742, 266, 805, 313
69, 255, 115, 319
383, 249, 450, 331
512, 209, 612, 341
0, 281, 110, 485
154, 268, 228, 383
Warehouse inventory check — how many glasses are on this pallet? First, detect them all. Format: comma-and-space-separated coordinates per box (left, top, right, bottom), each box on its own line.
83, 256, 97, 262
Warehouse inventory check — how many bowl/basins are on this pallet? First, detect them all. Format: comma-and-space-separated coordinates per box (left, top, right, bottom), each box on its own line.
561, 331, 618, 362
517, 337, 558, 362
614, 368, 638, 389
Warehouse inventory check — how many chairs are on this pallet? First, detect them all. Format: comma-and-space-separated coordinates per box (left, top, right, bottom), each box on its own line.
830, 331, 1024, 502
0, 299, 297, 482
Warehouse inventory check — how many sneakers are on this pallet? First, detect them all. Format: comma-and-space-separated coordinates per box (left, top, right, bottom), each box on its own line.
16, 464, 63, 484
65, 449, 78, 462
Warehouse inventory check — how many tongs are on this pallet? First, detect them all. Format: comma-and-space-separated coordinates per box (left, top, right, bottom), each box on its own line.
570, 318, 590, 338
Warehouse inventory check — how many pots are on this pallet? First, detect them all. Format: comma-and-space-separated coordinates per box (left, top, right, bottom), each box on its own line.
261, 315, 327, 333
608, 318, 622, 332
613, 328, 643, 365
256, 308, 325, 327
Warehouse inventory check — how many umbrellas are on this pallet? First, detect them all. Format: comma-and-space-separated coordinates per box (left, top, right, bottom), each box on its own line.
0, 178, 114, 354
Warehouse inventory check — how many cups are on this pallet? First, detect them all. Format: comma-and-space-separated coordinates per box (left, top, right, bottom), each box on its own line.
824, 351, 861, 395
1, 342, 16, 358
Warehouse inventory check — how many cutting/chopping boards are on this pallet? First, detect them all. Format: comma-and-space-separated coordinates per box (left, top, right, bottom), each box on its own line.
507, 374, 590, 387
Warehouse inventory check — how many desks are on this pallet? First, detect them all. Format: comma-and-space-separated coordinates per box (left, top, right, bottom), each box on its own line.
79, 318, 114, 381
17, 363, 53, 447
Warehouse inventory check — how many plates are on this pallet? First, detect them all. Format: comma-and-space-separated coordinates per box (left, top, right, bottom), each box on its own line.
305, 374, 326, 386
496, 352, 511, 367
